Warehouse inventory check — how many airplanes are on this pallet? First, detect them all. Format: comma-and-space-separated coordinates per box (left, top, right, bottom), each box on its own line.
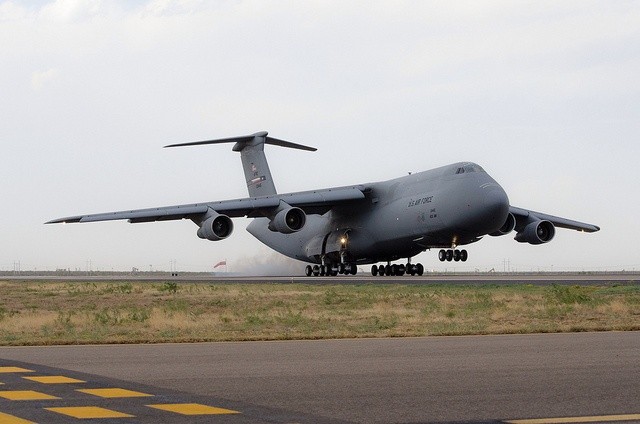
43, 131, 600, 276
